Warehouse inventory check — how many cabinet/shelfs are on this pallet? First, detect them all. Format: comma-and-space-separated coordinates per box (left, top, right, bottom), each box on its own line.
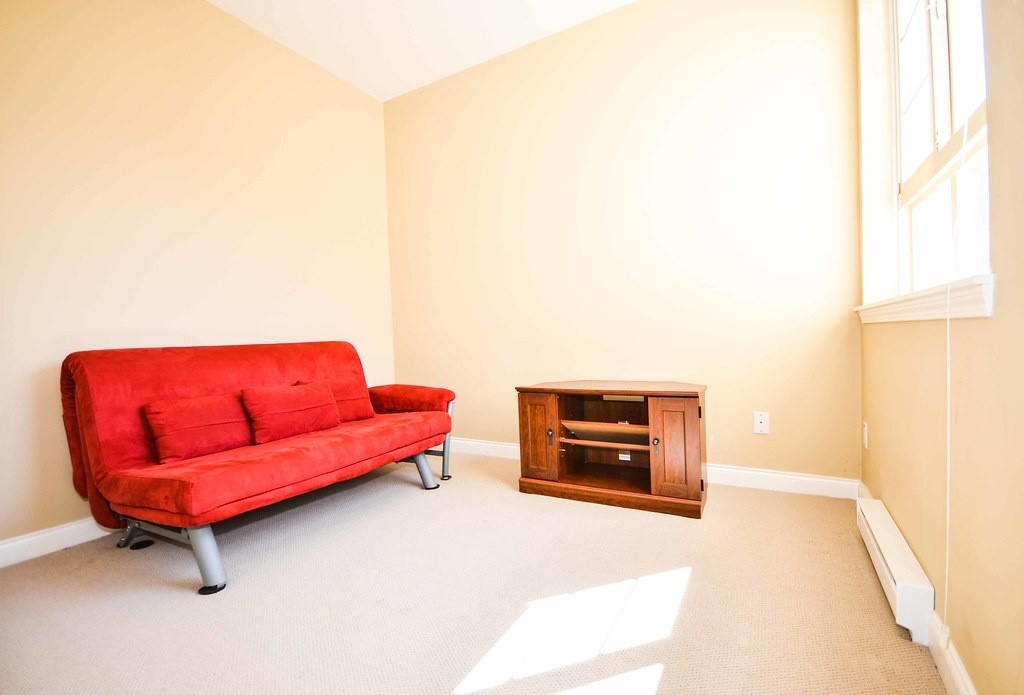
514, 379, 708, 520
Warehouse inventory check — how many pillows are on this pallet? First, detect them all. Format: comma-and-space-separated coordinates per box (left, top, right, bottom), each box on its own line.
240, 380, 341, 445
141, 395, 253, 465
295, 374, 378, 422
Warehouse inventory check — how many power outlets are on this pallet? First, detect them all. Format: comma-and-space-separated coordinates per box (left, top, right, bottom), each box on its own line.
753, 411, 770, 435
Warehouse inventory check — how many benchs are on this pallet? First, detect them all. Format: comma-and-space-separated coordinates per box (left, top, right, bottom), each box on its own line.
59, 339, 457, 595
